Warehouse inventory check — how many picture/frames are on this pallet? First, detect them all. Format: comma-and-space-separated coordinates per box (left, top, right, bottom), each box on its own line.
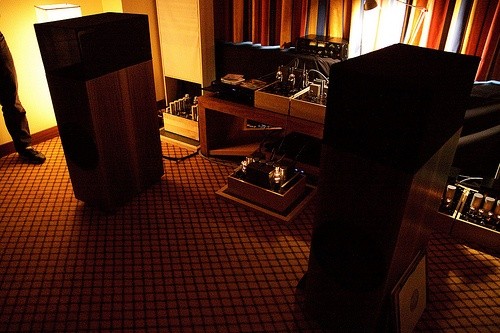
392, 248, 430, 333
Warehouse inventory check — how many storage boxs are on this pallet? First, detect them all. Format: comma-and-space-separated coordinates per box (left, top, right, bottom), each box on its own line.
228, 161, 303, 212
254, 81, 325, 124
439, 182, 499, 252
164, 110, 200, 140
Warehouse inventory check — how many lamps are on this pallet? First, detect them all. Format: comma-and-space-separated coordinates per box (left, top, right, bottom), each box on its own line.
35, 4, 82, 23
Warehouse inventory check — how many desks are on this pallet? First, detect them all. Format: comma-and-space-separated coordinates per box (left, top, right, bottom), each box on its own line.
197, 97, 324, 158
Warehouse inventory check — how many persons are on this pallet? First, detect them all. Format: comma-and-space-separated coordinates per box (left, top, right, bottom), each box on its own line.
0, 30, 46, 164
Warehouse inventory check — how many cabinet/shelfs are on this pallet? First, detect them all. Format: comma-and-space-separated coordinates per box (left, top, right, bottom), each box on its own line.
295, 44, 481, 333
35, 13, 165, 213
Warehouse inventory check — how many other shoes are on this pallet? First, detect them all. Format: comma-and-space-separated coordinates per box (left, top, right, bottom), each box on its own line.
19, 149, 47, 164
